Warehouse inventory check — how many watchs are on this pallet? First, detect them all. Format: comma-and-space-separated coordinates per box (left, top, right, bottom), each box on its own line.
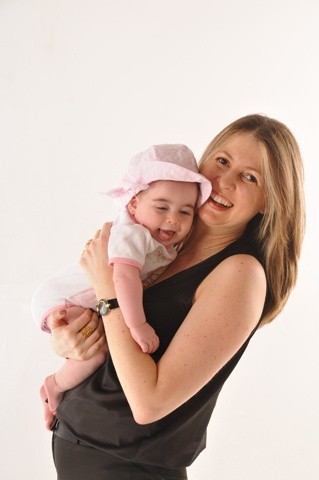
96, 297, 121, 318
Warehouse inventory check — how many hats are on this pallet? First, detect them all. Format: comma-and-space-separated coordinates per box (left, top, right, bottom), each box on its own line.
103, 144, 213, 211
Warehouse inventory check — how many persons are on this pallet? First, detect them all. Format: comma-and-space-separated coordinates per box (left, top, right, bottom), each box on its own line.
37, 140, 213, 435
51, 114, 305, 478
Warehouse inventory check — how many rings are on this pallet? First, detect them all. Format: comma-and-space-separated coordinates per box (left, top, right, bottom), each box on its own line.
80, 327, 95, 338
